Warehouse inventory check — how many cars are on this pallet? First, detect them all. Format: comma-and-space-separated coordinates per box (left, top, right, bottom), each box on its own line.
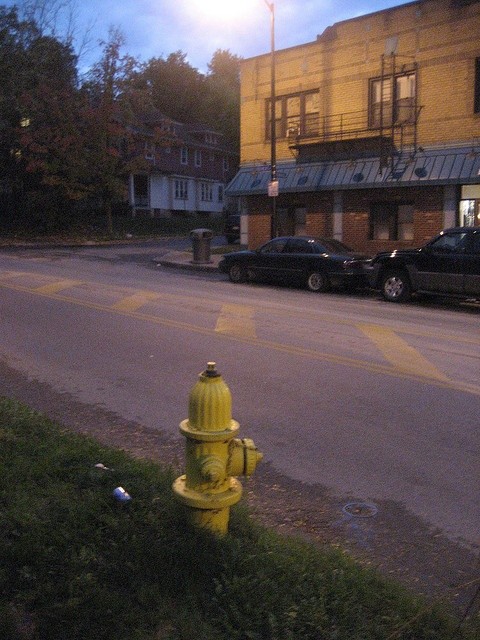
217, 236, 372, 292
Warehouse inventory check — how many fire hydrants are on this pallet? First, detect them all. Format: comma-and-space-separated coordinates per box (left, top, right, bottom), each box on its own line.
172, 362, 262, 539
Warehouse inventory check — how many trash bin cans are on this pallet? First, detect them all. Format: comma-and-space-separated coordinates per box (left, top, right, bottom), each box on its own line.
190, 228, 212, 263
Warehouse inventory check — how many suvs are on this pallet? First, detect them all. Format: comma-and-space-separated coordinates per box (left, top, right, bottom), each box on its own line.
370, 228, 480, 302
